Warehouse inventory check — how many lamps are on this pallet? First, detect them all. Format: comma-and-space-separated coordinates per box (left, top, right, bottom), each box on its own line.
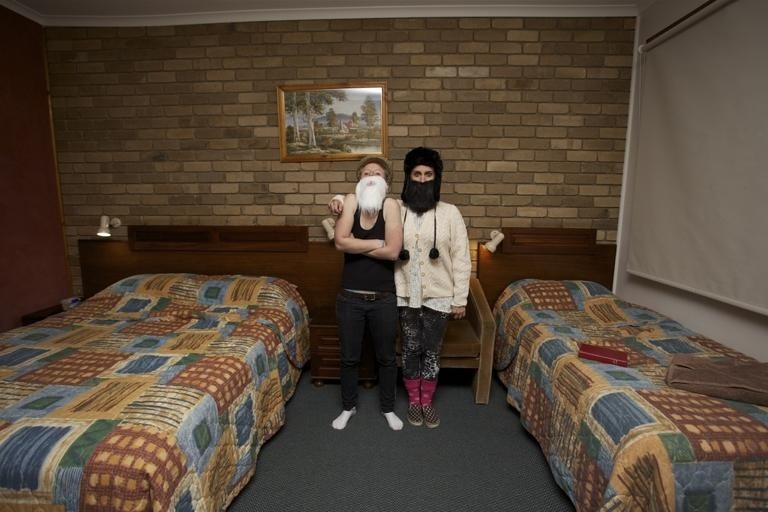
96, 214, 119, 238
485, 229, 505, 254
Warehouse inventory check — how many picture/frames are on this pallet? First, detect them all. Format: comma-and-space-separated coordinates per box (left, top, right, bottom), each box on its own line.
278, 81, 391, 164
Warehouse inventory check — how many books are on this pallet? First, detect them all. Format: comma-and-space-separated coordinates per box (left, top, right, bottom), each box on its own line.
577, 344, 631, 368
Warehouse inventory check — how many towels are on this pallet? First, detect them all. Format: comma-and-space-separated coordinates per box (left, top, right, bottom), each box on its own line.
665, 353, 768, 406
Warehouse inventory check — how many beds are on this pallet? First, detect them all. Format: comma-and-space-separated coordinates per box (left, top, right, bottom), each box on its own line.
479, 225, 768, 512
0, 224, 310, 511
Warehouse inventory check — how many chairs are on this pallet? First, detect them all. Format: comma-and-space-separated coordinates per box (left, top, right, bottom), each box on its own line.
439, 277, 497, 406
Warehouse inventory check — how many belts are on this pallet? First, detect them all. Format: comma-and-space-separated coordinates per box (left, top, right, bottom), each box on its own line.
338, 288, 396, 303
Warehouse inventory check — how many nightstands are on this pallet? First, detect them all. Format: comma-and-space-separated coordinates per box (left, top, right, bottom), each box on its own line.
310, 310, 343, 387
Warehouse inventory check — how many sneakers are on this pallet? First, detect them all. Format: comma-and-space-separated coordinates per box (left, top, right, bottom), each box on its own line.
421, 403, 441, 429
406, 401, 424, 427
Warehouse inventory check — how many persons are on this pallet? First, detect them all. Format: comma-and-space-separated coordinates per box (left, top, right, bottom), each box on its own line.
329, 146, 473, 430
326, 154, 403, 431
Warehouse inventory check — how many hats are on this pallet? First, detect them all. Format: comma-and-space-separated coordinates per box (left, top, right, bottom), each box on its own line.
401, 145, 444, 203
354, 154, 394, 185
